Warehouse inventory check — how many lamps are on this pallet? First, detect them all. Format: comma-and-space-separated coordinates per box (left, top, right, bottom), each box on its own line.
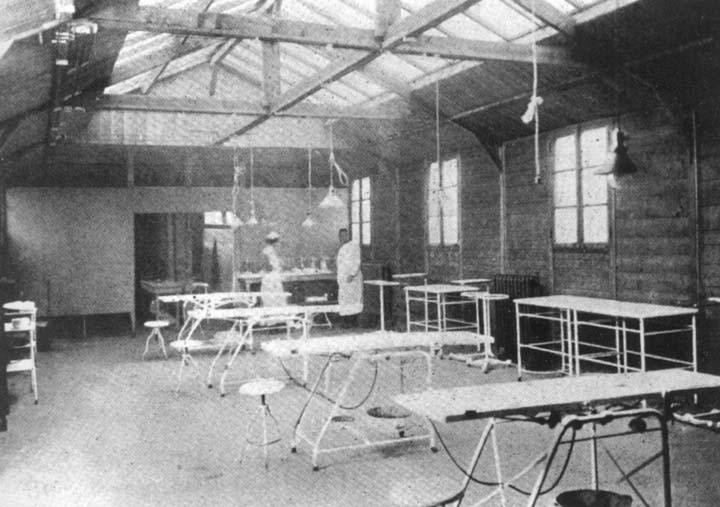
595, 0, 640, 187
319, 115, 352, 210
301, 132, 320, 230
230, 122, 258, 229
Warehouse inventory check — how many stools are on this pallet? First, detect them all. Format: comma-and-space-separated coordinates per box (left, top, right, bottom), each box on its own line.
387, 469, 465, 505
238, 377, 288, 467
139, 259, 512, 390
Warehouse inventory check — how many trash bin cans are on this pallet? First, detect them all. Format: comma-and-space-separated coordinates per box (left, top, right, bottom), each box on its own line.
556, 489, 632, 506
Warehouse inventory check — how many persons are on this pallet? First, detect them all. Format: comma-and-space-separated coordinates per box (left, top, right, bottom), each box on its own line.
336, 228, 364, 328
258, 231, 288, 335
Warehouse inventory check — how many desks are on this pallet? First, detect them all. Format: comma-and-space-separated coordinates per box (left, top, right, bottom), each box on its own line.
387, 365, 720, 506
2, 298, 47, 409
571, 300, 702, 407
261, 330, 496, 472
513, 292, 620, 428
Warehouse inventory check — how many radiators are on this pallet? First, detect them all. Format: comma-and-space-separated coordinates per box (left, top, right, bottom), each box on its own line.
489, 268, 548, 371
